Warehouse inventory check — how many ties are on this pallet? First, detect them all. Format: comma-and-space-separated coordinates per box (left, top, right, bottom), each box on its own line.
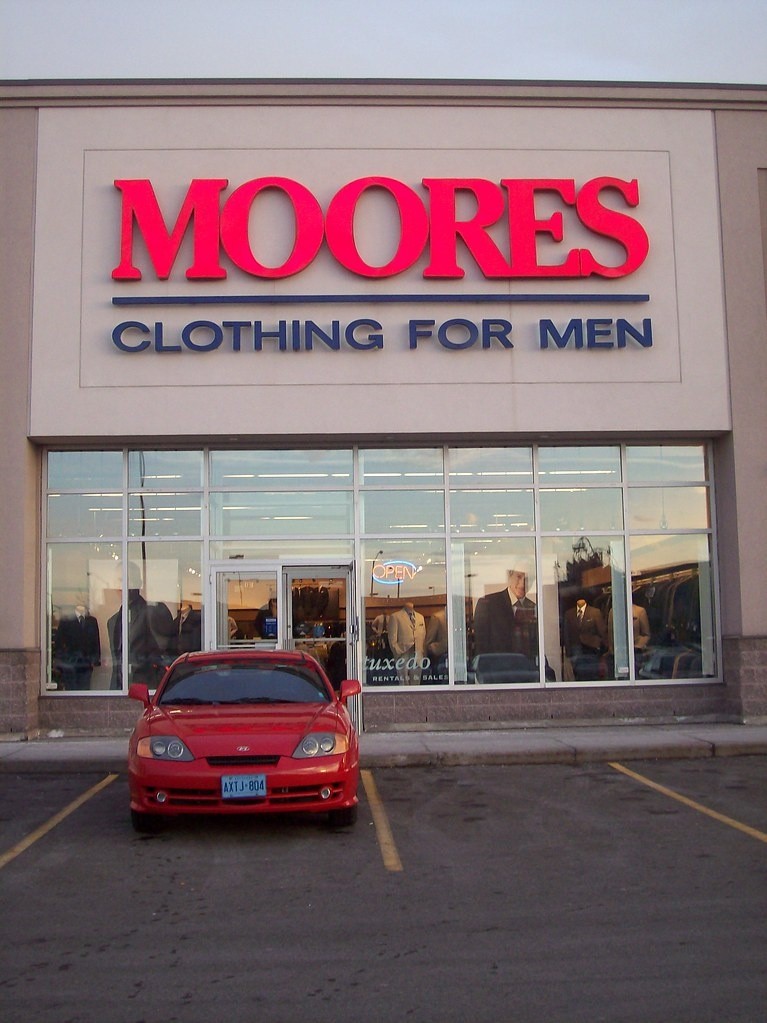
180, 617, 184, 623
383, 616, 387, 631
577, 610, 582, 632
79, 615, 85, 631
513, 601, 531, 658
410, 612, 415, 627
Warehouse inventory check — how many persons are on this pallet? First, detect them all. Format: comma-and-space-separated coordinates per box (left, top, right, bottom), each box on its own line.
474, 555, 538, 668
172, 605, 201, 656
255, 594, 298, 640
607, 601, 650, 679
53, 605, 101, 691
562, 599, 610, 682
106, 561, 176, 691
228, 616, 238, 637
371, 610, 392, 666
388, 601, 428, 686
427, 605, 450, 684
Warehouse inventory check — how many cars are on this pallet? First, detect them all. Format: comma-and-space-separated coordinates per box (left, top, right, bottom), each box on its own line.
468, 653, 540, 686
128, 650, 363, 835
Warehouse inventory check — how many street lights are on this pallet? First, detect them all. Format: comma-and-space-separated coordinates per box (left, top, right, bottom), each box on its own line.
370, 550, 383, 597
429, 586, 435, 595
